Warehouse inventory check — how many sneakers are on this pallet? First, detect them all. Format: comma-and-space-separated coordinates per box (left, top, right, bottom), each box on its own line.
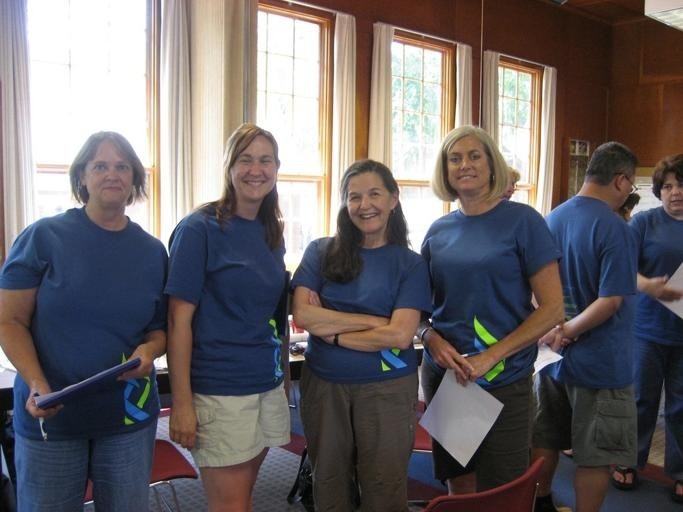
553, 506, 573, 512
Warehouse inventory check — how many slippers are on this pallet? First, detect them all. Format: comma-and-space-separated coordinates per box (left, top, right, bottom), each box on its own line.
610, 463, 637, 492
670, 480, 683, 503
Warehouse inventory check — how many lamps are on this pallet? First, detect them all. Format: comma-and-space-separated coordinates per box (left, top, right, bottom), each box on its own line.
644, 0, 683, 32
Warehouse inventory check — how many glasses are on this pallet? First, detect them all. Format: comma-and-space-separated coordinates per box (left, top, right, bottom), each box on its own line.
613, 170, 638, 196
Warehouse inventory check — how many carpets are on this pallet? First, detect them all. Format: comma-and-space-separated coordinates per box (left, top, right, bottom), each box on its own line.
639, 390, 675, 486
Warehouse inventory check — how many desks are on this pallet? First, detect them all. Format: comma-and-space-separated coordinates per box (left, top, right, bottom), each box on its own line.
0, 343, 428, 511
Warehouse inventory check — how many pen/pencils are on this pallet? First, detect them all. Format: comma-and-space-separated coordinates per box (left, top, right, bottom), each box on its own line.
459, 352, 480, 357
33, 392, 48, 442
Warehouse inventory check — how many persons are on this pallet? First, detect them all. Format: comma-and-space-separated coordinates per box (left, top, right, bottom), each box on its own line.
0, 130, 169, 512
163, 120, 292, 512
529, 138, 638, 512
285, 158, 432, 512
414, 123, 565, 496
609, 152, 682, 504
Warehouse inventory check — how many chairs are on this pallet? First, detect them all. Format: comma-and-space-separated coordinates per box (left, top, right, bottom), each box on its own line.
417, 451, 545, 512
84, 436, 199, 510
255, 430, 312, 506
406, 401, 436, 454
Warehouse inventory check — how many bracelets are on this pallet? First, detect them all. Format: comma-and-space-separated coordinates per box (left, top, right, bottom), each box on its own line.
420, 326, 428, 344
333, 333, 339, 346
